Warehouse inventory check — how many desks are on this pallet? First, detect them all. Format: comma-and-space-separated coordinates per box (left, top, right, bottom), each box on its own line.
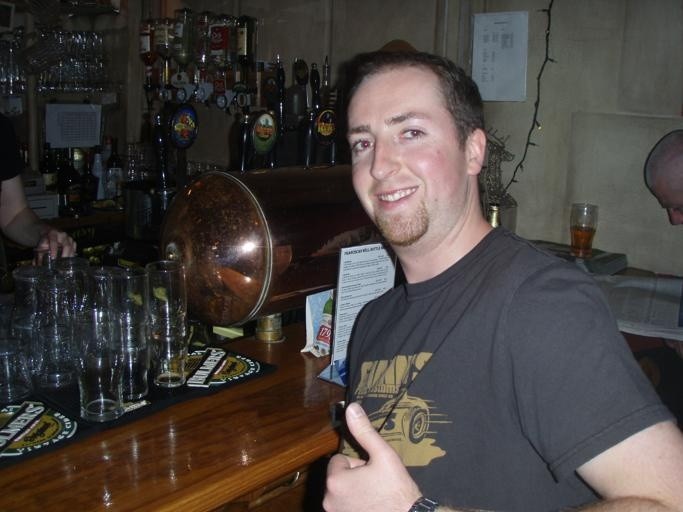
0, 330, 346, 511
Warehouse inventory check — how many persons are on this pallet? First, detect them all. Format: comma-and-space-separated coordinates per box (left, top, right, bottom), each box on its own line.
316, 37, 683, 512
0, 136, 79, 261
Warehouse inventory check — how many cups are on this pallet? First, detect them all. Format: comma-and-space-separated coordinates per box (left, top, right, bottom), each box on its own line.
570, 202, 599, 259
0, 28, 107, 97
0, 255, 191, 423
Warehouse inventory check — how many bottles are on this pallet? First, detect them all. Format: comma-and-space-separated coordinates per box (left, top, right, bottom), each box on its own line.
185, 162, 218, 185
138, 7, 260, 91
487, 201, 502, 230
236, 54, 338, 172
14, 113, 175, 218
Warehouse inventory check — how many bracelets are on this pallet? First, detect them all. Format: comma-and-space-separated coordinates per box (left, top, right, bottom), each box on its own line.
408, 495, 440, 512
